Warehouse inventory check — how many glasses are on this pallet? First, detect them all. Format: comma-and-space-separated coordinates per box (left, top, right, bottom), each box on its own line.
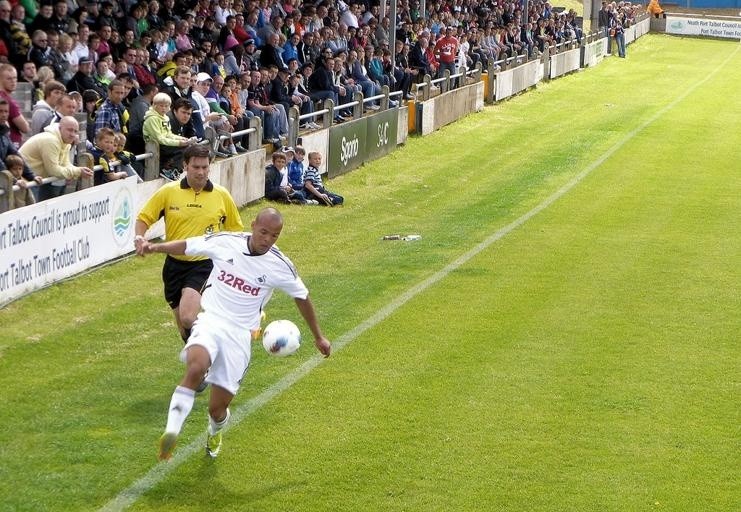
326, 52, 331, 53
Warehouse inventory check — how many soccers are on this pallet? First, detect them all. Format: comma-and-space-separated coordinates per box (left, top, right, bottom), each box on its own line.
264, 320, 301, 357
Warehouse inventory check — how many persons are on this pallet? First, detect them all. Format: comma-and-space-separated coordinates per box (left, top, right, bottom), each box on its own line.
2, 0, 386, 208
645, 1, 665, 15
599, 2, 643, 58
137, 208, 330, 462
368, 1, 584, 105
133, 145, 244, 393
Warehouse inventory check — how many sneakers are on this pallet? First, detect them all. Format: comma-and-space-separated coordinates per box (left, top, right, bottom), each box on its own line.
265, 121, 320, 144
333, 91, 415, 124
227, 143, 237, 155
159, 168, 177, 183
321, 195, 333, 207
156, 431, 178, 465
235, 141, 246, 152
305, 199, 319, 205
172, 168, 180, 181
206, 426, 223, 458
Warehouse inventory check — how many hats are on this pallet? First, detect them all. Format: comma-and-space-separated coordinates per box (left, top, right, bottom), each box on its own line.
242, 39, 254, 47
196, 72, 212, 85
281, 146, 295, 155
225, 35, 239, 49
78, 56, 93, 65
